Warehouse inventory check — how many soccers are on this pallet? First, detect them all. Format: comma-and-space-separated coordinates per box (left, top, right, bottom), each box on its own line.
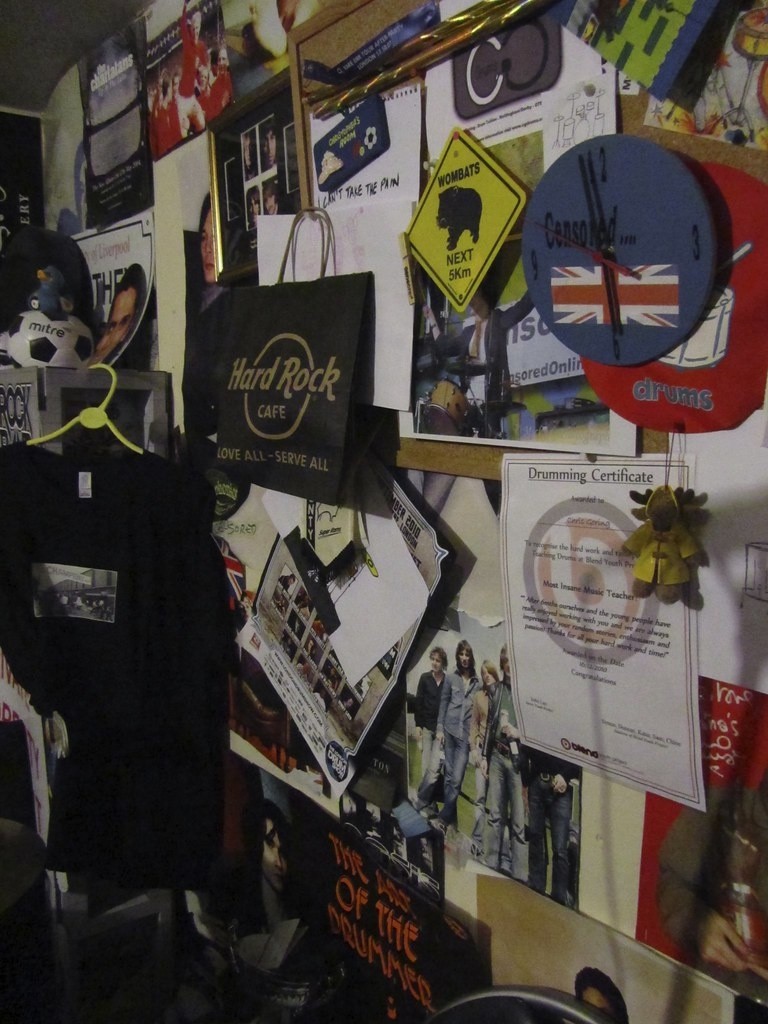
7, 309, 94, 368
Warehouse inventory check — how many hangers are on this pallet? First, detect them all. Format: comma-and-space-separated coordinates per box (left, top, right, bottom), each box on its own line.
0, 363, 216, 506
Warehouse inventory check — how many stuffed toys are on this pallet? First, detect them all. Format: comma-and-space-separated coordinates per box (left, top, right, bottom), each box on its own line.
28, 264, 73, 323
621, 482, 709, 605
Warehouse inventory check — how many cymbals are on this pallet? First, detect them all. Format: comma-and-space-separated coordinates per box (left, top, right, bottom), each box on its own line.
443, 356, 486, 377
479, 400, 528, 416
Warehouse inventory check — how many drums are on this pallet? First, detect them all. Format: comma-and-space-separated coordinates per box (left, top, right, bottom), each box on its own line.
419, 377, 468, 433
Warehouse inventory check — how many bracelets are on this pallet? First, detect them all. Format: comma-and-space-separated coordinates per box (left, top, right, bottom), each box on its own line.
430, 322, 438, 329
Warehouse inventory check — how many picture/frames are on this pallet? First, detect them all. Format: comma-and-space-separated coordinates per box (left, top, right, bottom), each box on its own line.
206, 66, 311, 284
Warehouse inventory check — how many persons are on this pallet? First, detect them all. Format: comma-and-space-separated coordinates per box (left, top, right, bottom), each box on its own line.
220, 798, 307, 968
56, 587, 115, 622
469, 659, 513, 875
421, 266, 536, 439
88, 264, 145, 368
659, 764, 767, 1007
146, 1, 283, 232
478, 641, 533, 886
429, 641, 482, 836
575, 966, 629, 1024
272, 562, 398, 725
199, 191, 223, 315
411, 646, 449, 818
520, 742, 579, 909
